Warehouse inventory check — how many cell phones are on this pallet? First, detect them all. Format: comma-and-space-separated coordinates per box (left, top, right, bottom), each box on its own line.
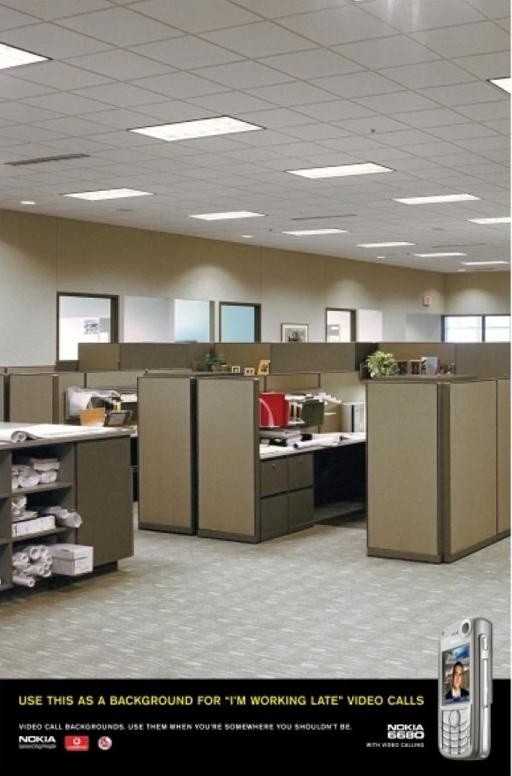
438, 616, 494, 762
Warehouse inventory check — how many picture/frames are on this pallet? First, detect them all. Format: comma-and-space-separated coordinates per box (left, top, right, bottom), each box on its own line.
281, 323, 308, 343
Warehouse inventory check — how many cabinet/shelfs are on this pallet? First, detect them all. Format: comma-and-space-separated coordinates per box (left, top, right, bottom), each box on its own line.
0, 419, 135, 591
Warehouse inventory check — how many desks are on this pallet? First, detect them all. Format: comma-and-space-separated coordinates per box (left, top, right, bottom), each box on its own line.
260, 430, 363, 542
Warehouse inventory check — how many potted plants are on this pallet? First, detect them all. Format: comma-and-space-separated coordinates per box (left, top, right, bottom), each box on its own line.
364, 350, 399, 379
209, 355, 228, 371
